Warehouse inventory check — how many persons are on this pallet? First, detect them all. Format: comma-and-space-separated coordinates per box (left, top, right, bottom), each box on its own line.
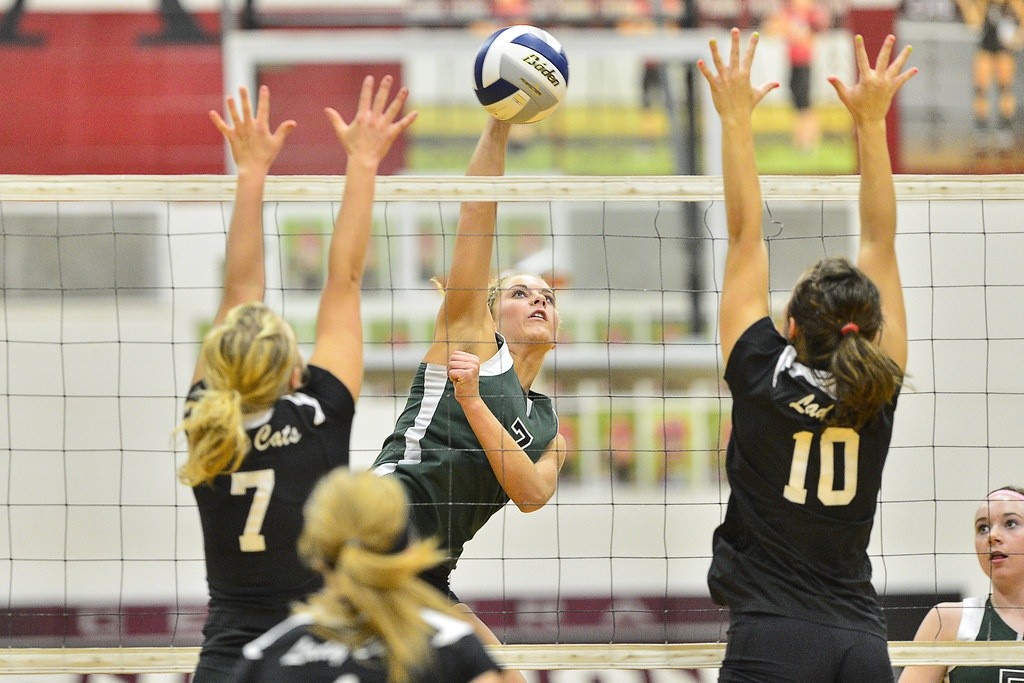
696, 24, 921, 683
188, 463, 533, 682
172, 74, 420, 681
360, 116, 571, 682
896, 486, 1024, 683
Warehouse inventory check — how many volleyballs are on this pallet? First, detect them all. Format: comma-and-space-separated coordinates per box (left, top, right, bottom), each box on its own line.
474, 22, 570, 124
